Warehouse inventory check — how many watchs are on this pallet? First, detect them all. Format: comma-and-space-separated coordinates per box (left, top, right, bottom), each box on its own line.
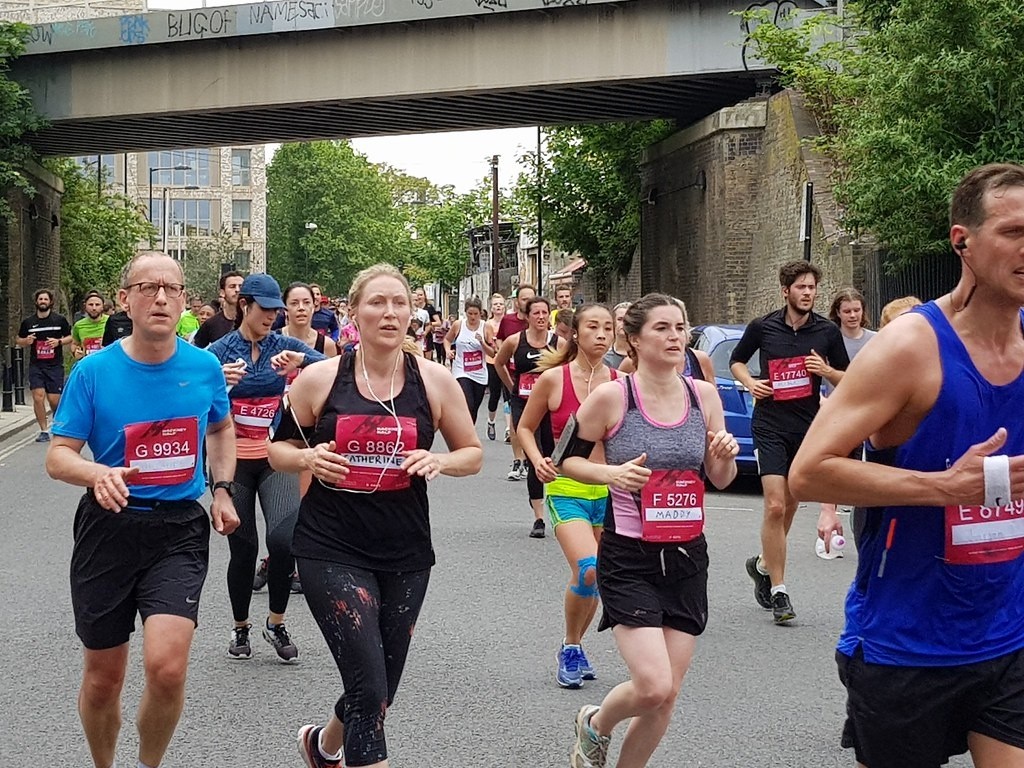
214, 480, 236, 497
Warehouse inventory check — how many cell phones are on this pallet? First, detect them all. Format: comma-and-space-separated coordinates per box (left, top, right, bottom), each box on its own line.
224, 358, 247, 394
550, 414, 578, 465
268, 394, 290, 440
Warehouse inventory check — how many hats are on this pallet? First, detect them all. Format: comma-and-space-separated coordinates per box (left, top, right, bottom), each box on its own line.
240, 272, 289, 311
321, 296, 328, 303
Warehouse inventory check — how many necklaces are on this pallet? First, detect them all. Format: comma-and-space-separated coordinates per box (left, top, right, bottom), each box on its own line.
574, 361, 603, 384
285, 327, 310, 346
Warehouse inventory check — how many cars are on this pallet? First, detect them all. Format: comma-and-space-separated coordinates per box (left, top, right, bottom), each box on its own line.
688, 323, 829, 491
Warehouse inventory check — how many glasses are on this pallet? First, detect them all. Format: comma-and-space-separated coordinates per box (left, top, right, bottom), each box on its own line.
126, 282, 184, 299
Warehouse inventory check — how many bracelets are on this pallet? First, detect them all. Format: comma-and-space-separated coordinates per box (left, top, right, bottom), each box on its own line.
72, 348, 79, 357
57, 338, 61, 346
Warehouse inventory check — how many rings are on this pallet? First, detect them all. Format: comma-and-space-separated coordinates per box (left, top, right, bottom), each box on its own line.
728, 443, 734, 450
427, 464, 433, 470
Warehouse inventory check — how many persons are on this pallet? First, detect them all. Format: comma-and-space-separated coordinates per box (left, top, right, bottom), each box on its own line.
729, 260, 850, 622
264, 265, 482, 768
517, 300, 627, 688
550, 292, 739, 768
208, 272, 327, 662
78, 283, 375, 596
790, 163, 1024, 768
408, 267, 716, 537
817, 286, 923, 555
45, 252, 241, 768
15, 288, 72, 443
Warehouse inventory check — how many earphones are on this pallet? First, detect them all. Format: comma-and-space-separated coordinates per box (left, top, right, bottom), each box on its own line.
573, 333, 578, 338
954, 243, 967, 249
351, 316, 356, 320
244, 305, 249, 316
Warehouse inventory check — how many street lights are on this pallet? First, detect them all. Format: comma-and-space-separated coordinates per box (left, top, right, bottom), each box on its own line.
149, 166, 192, 249
161, 185, 201, 253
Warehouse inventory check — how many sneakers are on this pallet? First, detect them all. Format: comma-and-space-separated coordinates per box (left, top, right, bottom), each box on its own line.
815, 537, 844, 560
556, 637, 598, 687
745, 555, 772, 609
227, 623, 253, 659
297, 724, 343, 768
35, 432, 50, 442
504, 430, 511, 442
519, 459, 529, 479
529, 518, 546, 538
252, 556, 269, 591
508, 459, 522, 480
487, 422, 495, 440
571, 704, 611, 768
290, 574, 302, 594
770, 591, 797, 623
262, 616, 299, 662
